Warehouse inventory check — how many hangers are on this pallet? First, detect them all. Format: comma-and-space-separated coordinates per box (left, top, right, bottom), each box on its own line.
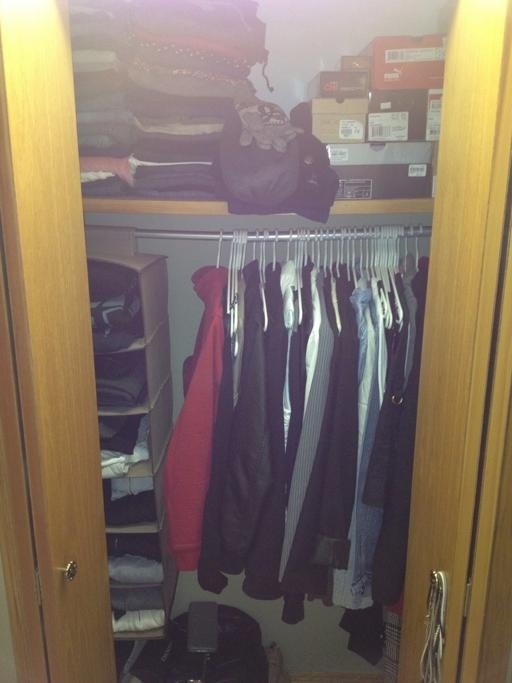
213, 222, 419, 337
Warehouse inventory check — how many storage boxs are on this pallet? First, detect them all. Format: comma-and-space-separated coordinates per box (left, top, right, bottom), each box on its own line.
291, 33, 447, 200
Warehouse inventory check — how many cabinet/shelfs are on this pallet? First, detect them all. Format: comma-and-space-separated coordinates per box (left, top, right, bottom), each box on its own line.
86, 226, 179, 640
